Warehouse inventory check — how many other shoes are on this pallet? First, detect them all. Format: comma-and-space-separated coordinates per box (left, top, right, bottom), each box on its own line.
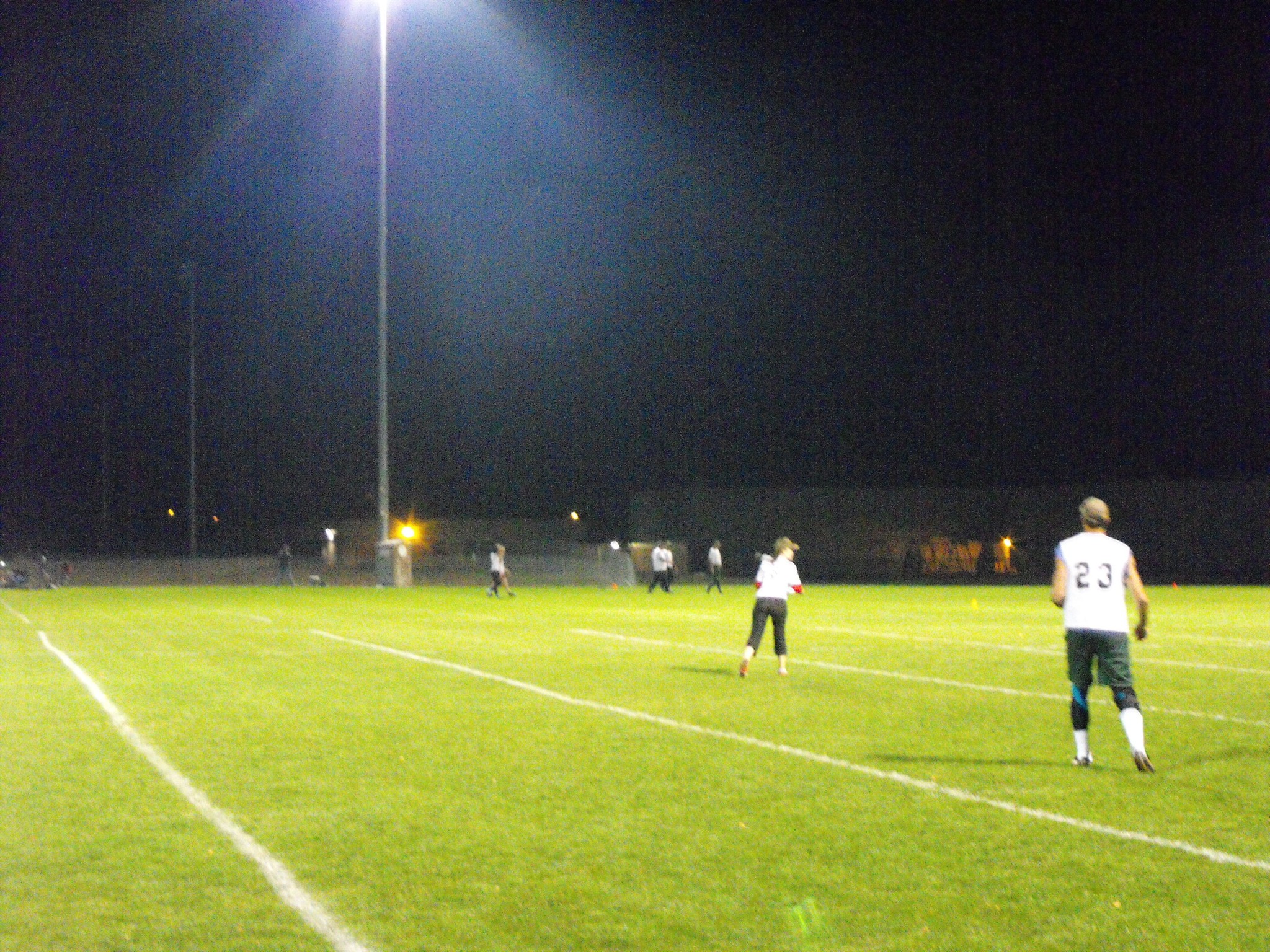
776, 668, 788, 676
1073, 752, 1090, 766
739, 659, 749, 678
1132, 749, 1156, 773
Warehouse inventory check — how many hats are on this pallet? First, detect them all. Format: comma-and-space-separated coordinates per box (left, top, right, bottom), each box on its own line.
773, 537, 800, 555
1079, 496, 1110, 525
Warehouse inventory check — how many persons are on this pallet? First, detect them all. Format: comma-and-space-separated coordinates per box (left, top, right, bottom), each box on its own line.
646, 539, 675, 595
739, 537, 803, 679
274, 542, 296, 588
1050, 496, 1157, 774
486, 543, 517, 601
706, 540, 723, 595
